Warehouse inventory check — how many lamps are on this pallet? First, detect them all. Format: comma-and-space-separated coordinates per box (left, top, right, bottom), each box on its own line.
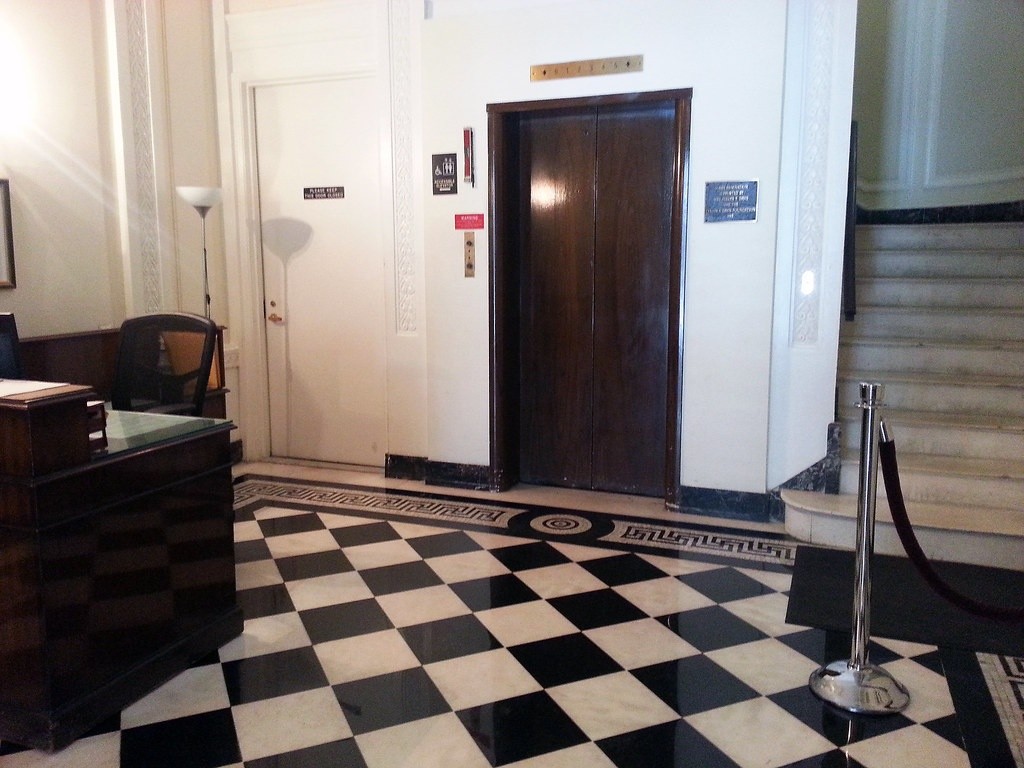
176, 186, 223, 317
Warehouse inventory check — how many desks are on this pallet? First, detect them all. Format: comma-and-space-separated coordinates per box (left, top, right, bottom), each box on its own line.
0, 377, 243, 755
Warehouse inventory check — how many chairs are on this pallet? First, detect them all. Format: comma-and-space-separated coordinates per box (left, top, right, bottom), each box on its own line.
110, 312, 216, 416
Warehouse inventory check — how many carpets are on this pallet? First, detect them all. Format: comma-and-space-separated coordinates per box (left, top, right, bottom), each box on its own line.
783, 544, 1024, 768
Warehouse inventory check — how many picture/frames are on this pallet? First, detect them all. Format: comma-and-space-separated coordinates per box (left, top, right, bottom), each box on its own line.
0, 179, 17, 288
